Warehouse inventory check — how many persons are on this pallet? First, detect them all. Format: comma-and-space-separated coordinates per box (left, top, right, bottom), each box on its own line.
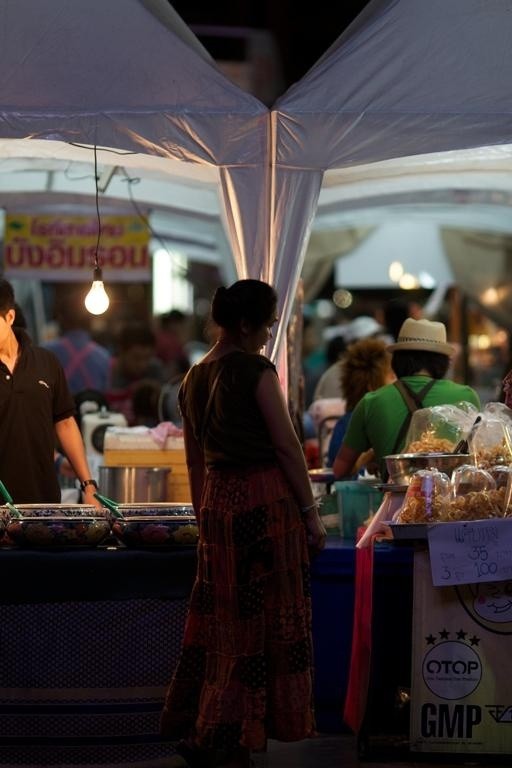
158, 279, 329, 768
329, 339, 398, 477
40, 300, 210, 466
333, 318, 480, 480
296, 300, 418, 468
0, 276, 105, 510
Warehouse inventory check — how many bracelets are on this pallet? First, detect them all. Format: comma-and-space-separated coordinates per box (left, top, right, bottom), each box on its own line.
80, 479, 98, 491
301, 502, 318, 515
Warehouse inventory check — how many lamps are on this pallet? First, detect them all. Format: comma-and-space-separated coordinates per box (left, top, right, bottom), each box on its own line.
84, 144, 110, 315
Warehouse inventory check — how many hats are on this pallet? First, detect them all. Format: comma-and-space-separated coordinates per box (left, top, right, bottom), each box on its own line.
386, 318, 457, 359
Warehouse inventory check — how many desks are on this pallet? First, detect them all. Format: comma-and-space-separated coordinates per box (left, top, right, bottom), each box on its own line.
0, 520, 512, 768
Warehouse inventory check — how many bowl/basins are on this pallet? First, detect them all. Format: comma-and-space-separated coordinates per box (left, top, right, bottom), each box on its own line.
381, 451, 473, 485
113, 514, 199, 548
0, 503, 109, 547
109, 502, 195, 525
